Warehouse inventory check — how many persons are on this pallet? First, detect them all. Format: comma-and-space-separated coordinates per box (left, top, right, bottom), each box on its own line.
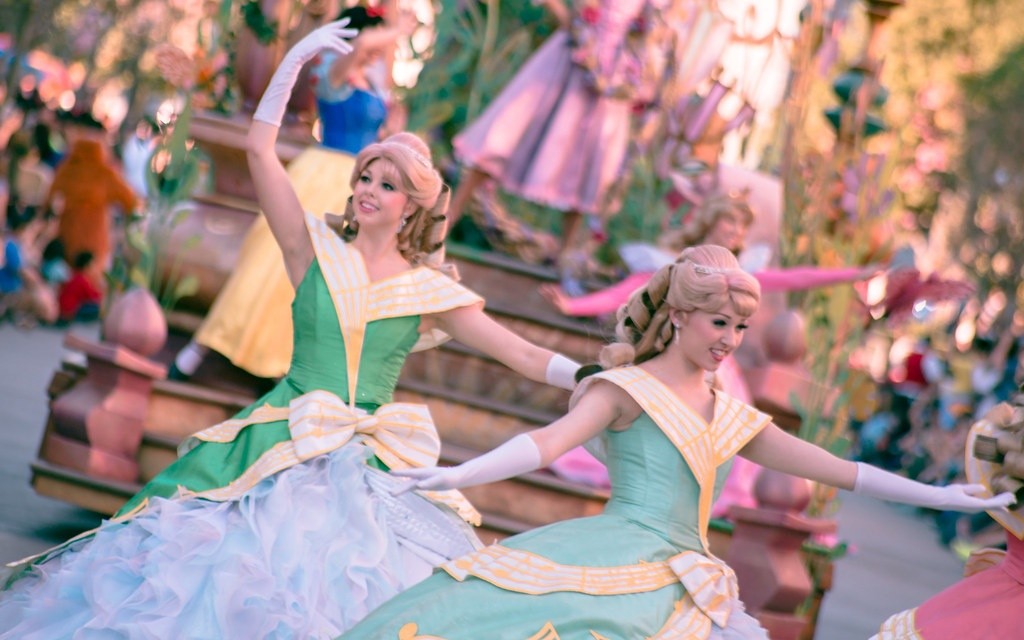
446, 0, 673, 294
165, 7, 414, 380
0, 16, 583, 640
0, 112, 147, 329
335, 245, 1017, 640
537, 194, 880, 511
